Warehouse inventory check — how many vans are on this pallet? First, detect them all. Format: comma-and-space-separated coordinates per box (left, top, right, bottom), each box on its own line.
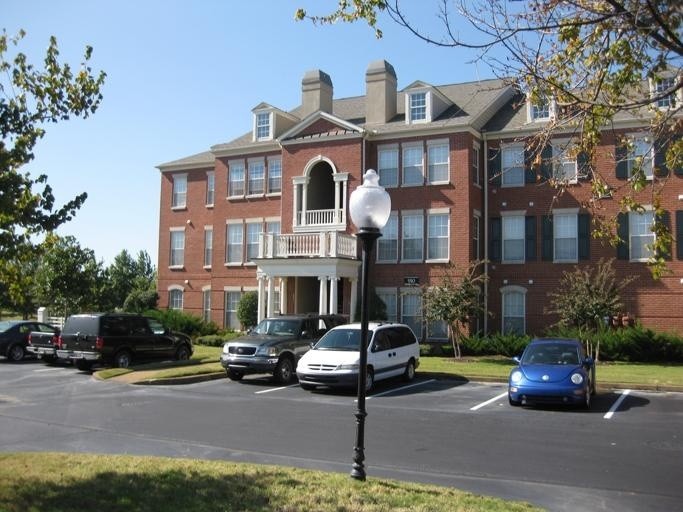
296, 321, 420, 396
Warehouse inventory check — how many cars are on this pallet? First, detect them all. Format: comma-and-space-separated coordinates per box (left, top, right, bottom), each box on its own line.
0, 313, 194, 371
221, 313, 347, 384
509, 338, 596, 410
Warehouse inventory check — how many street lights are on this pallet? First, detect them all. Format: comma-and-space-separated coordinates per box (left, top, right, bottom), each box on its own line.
348, 168, 392, 480
